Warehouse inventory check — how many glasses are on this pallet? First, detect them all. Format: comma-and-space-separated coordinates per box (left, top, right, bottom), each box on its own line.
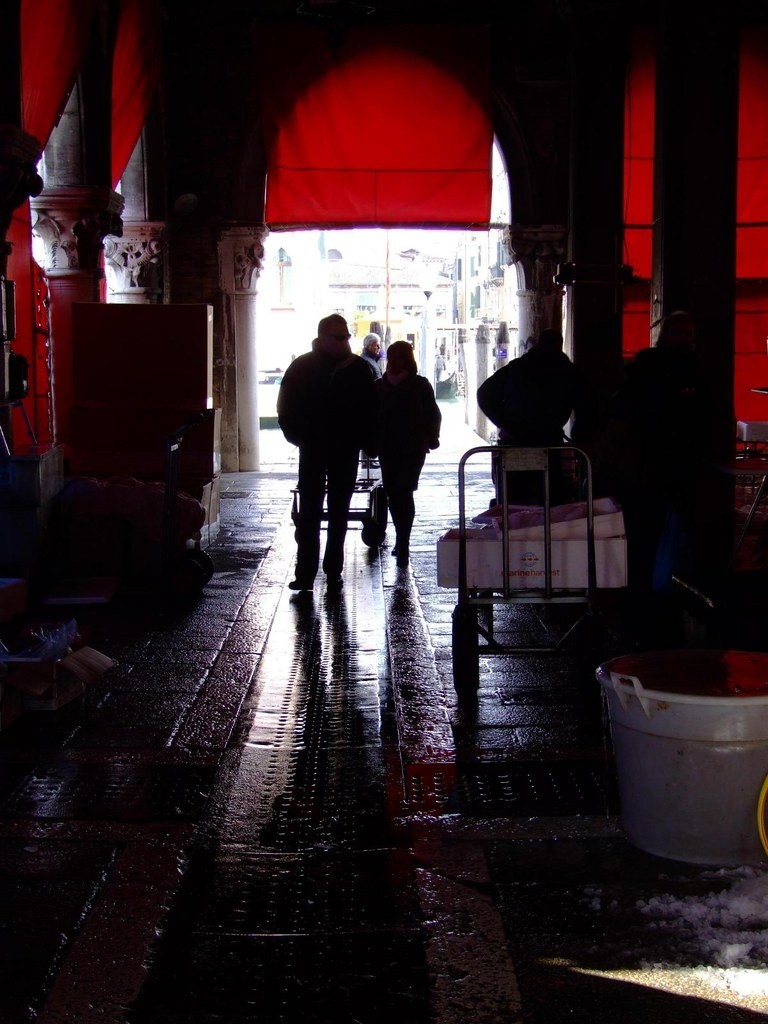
324, 334, 351, 341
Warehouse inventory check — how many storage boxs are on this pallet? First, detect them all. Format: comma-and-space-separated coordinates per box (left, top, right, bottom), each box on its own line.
436, 495, 629, 588
183, 473, 221, 524
0, 636, 117, 711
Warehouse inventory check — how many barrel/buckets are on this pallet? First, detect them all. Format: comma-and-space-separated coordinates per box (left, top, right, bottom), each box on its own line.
595, 656, 767, 867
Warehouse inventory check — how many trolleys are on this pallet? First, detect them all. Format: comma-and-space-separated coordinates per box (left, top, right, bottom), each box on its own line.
45, 434, 214, 604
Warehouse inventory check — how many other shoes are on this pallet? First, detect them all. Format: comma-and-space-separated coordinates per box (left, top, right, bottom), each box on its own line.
326, 575, 343, 583
288, 580, 312, 590
361, 459, 381, 469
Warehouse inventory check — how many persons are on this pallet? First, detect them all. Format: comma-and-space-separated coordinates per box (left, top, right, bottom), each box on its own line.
277, 314, 378, 589
369, 340, 441, 557
474, 325, 711, 579
308, 323, 395, 471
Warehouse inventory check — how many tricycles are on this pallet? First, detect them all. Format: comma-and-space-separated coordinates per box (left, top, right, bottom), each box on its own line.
290, 449, 390, 547
452, 436, 604, 701
